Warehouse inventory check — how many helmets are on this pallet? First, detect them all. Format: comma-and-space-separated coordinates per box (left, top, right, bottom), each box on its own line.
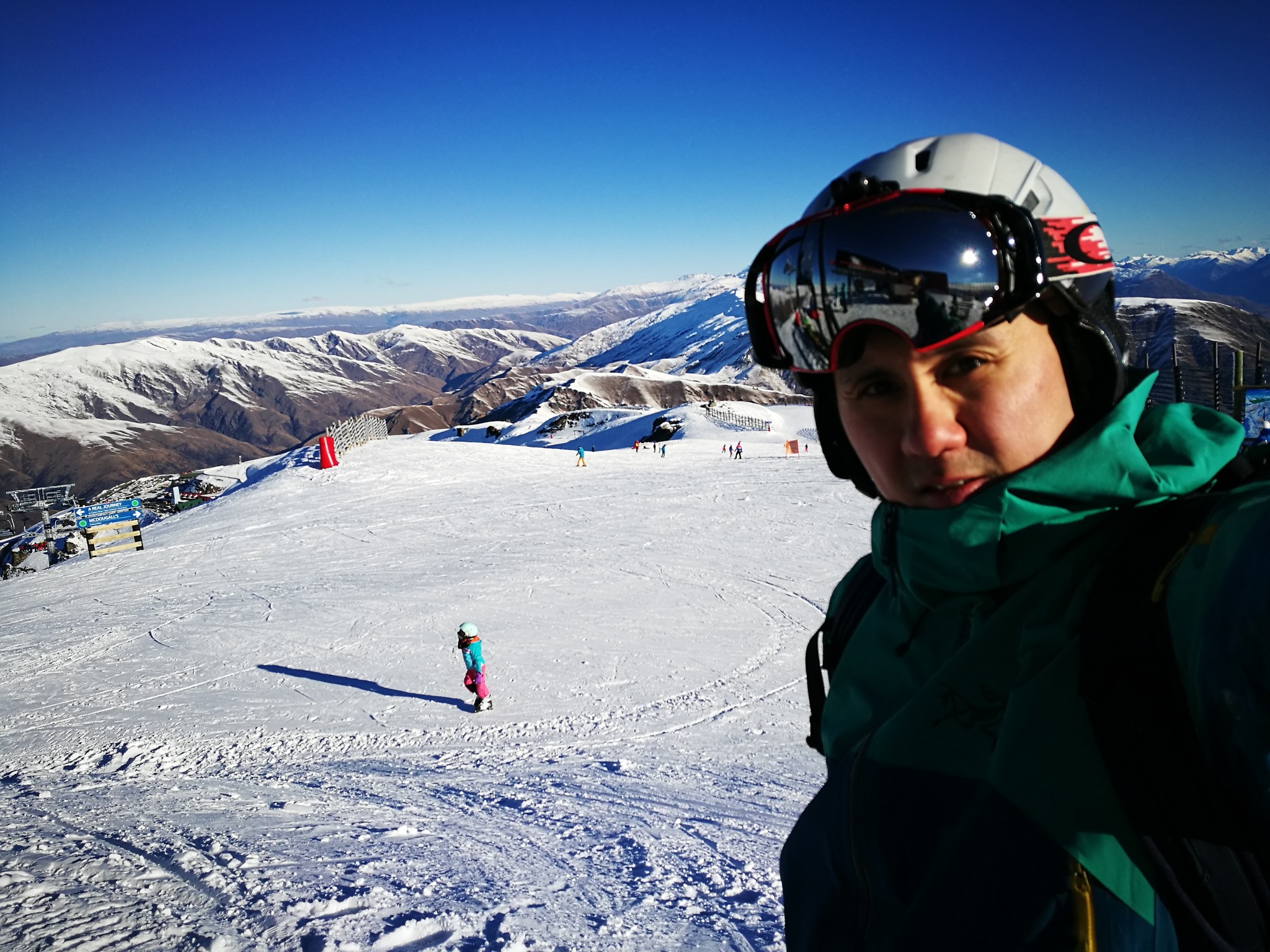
457, 622, 478, 643
799, 132, 1128, 422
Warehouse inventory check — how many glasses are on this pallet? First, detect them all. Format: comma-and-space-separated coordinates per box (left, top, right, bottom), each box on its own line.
744, 188, 1053, 374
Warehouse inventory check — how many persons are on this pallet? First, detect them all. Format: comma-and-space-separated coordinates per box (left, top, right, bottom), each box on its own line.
457, 622, 493, 713
659, 444, 661, 452
661, 444, 666, 458
729, 445, 733, 456
805, 444, 808, 452
636, 440, 638, 452
33, 539, 47, 552
19, 542, 29, 552
653, 444, 656, 452
576, 446, 586, 467
722, 445, 726, 453
833, 282, 848, 314
735, 443, 743, 459
745, 132, 1270, 952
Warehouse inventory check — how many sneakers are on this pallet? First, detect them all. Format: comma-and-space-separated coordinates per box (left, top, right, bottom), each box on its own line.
473, 694, 494, 713
